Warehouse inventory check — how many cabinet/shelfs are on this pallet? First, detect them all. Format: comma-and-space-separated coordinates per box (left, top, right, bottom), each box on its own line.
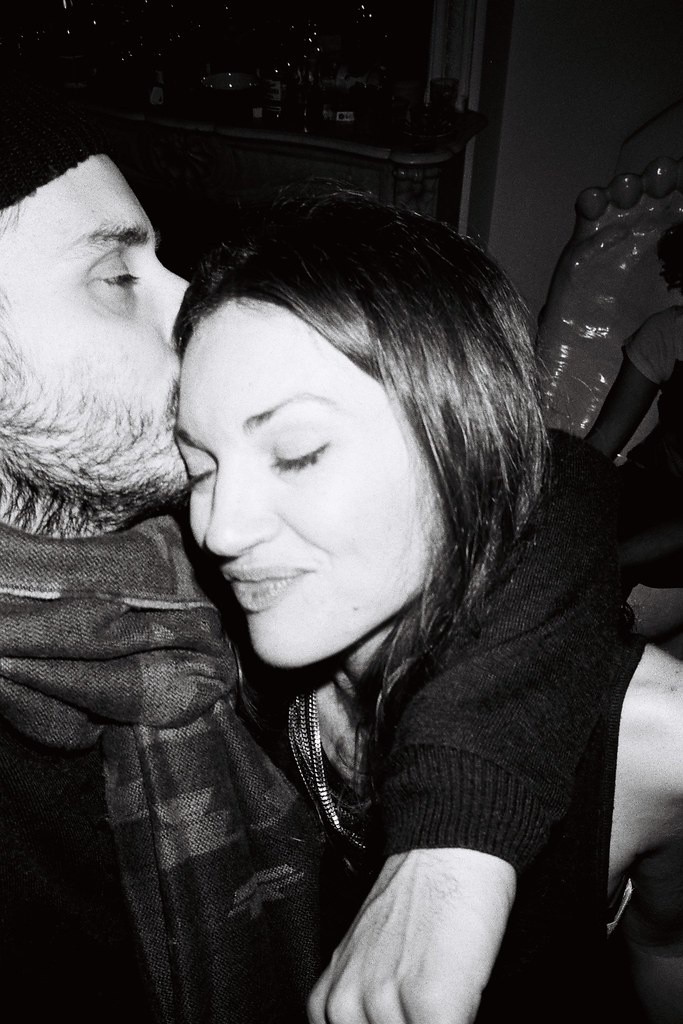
68, 85, 483, 244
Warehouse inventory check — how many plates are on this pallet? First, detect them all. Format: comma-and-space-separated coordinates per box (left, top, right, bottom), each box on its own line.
390, 104, 455, 140
201, 72, 257, 90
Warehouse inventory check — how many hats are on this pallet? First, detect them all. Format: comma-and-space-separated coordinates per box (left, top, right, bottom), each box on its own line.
0, 84, 118, 210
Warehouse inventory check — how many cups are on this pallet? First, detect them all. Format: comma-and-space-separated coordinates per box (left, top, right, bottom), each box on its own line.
429, 76, 458, 120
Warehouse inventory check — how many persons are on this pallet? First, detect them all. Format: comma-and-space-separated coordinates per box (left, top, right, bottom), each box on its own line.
177, 195, 683, 1023
0, 123, 683, 1023
583, 218, 683, 475
